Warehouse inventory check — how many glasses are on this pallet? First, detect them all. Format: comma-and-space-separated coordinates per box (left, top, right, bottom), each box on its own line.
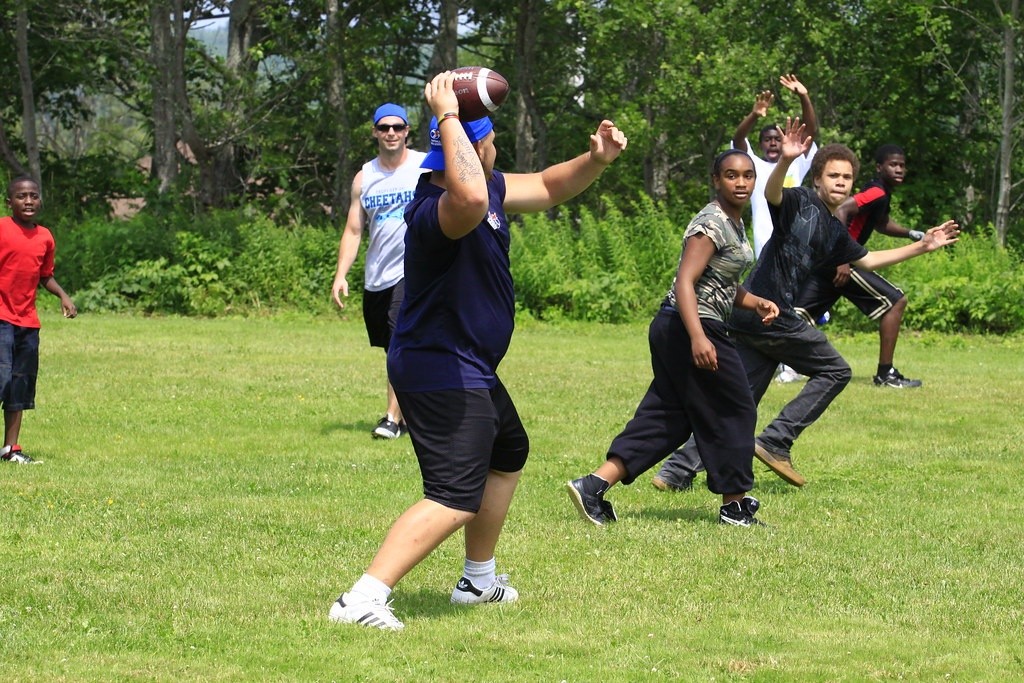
375, 123, 406, 132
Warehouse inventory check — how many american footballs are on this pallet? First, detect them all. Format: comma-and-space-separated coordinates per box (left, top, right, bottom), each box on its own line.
448, 67, 510, 123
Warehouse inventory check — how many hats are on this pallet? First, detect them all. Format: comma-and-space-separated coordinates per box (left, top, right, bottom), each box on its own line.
374, 103, 407, 127
419, 114, 492, 171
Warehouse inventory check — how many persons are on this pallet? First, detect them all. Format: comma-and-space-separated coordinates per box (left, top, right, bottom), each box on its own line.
331, 102, 436, 438
564, 145, 780, 529
793, 144, 927, 389
730, 73, 819, 383
0, 175, 77, 465
325, 71, 628, 632
651, 115, 961, 493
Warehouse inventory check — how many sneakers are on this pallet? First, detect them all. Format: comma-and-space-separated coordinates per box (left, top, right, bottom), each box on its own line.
450, 574, 518, 604
755, 442, 805, 486
398, 420, 408, 433
720, 496, 766, 527
329, 591, 404, 631
652, 477, 675, 491
568, 474, 617, 525
0, 444, 35, 463
873, 367, 922, 388
374, 414, 402, 438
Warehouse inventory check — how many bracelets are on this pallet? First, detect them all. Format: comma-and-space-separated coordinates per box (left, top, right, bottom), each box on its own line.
437, 112, 459, 127
